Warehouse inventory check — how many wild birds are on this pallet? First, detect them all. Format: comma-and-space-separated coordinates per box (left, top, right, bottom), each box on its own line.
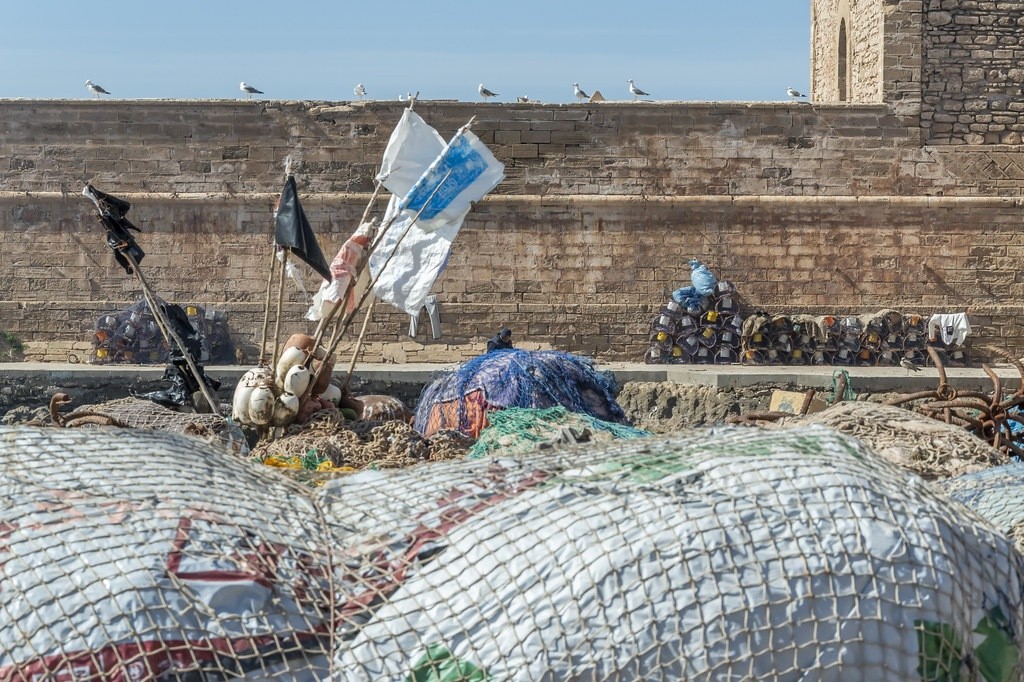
84, 80, 111, 99
237, 81, 264, 99
516, 94, 529, 103
625, 79, 651, 100
570, 80, 592, 104
477, 83, 500, 102
354, 83, 368, 99
784, 87, 808, 101
